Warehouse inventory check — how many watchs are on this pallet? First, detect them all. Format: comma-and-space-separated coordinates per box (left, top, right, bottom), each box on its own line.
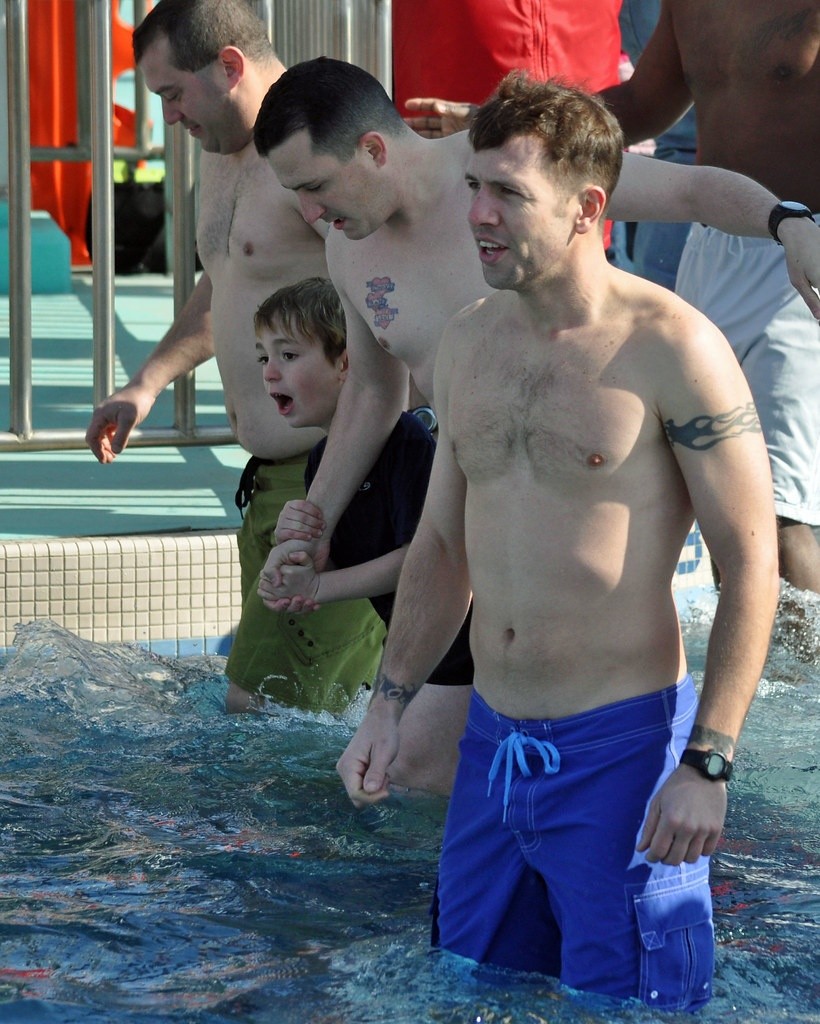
768, 200, 815, 247
679, 749, 735, 782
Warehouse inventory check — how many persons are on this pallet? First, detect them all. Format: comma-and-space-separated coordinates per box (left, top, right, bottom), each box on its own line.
336, 70, 777, 1015
84, 0, 820, 802
